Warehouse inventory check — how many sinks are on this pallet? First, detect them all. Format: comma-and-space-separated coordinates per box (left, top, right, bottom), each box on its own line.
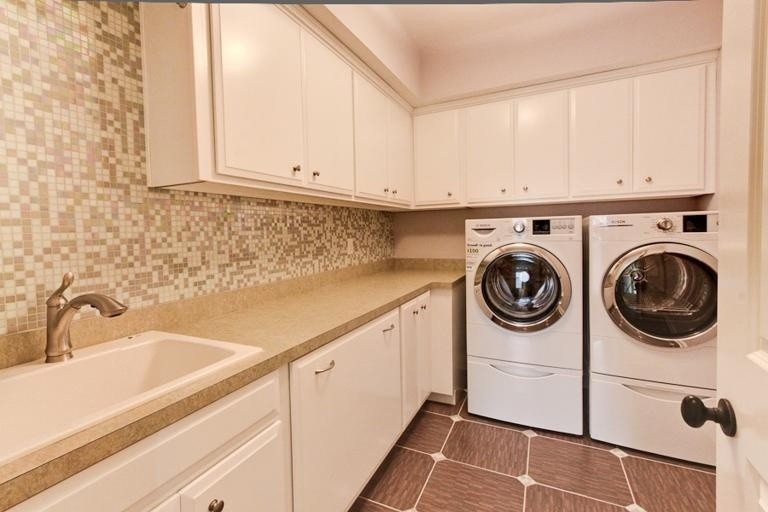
0, 330, 264, 469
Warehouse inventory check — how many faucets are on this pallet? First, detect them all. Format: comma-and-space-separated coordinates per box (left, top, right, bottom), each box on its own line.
43, 272, 128, 362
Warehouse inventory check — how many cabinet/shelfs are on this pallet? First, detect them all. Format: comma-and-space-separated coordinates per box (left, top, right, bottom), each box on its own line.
400, 291, 431, 434
290, 306, 402, 512
141, 3, 718, 210
431, 288, 465, 405
0, 370, 283, 512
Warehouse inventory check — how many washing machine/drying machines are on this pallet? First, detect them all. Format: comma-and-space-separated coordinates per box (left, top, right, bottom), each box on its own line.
464, 215, 583, 437
583, 212, 717, 469
